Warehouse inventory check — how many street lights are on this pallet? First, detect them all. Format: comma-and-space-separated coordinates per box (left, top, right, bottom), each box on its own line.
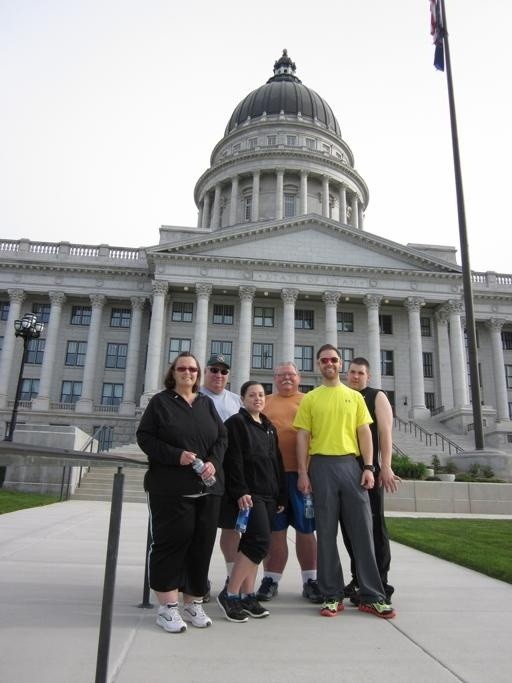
0, 312, 45, 489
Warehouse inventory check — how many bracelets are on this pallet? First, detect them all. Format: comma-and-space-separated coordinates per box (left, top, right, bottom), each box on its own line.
363, 465, 375, 472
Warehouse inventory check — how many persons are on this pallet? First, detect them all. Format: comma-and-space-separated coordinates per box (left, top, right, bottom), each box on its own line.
199, 355, 245, 603
256, 361, 325, 603
216, 381, 288, 623
343, 357, 402, 606
136, 352, 229, 634
290, 344, 397, 618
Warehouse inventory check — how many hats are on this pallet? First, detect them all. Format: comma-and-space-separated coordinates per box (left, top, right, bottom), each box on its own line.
207, 353, 231, 369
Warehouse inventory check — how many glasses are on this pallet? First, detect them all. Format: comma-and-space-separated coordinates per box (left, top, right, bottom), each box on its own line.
321, 357, 339, 364
177, 367, 198, 372
210, 367, 229, 375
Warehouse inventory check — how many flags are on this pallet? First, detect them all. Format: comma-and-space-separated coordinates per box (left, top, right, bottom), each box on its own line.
429, 0, 447, 72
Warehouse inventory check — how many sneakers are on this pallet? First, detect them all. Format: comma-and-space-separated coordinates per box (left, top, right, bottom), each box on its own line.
302, 578, 396, 619
157, 603, 189, 632
202, 581, 211, 603
179, 600, 212, 628
216, 577, 278, 622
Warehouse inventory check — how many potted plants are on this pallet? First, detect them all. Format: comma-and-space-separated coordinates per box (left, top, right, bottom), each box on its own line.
437, 462, 456, 482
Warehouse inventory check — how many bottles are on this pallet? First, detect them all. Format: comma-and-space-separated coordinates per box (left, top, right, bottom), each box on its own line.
234, 507, 251, 534
304, 493, 315, 519
191, 455, 217, 488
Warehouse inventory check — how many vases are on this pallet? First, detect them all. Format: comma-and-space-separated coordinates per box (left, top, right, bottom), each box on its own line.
422, 469, 434, 480
